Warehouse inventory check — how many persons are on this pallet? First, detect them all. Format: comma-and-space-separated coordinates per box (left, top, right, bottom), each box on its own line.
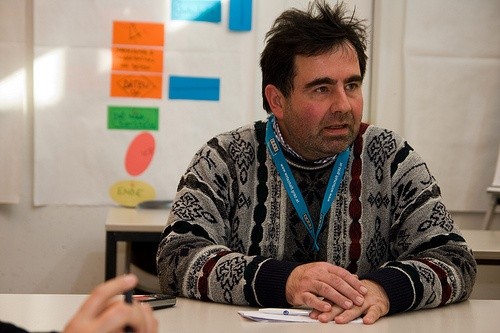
156, 0, 476, 324
0, 273, 158, 333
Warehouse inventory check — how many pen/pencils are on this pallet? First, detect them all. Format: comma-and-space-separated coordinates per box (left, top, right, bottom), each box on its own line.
123, 237, 132, 333
283, 310, 310, 316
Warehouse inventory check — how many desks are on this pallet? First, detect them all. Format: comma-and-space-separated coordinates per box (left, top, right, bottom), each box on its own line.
105, 209, 500, 281
0, 293, 500, 333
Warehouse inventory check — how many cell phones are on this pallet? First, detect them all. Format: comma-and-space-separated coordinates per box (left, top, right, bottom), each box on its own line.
132, 294, 176, 308
